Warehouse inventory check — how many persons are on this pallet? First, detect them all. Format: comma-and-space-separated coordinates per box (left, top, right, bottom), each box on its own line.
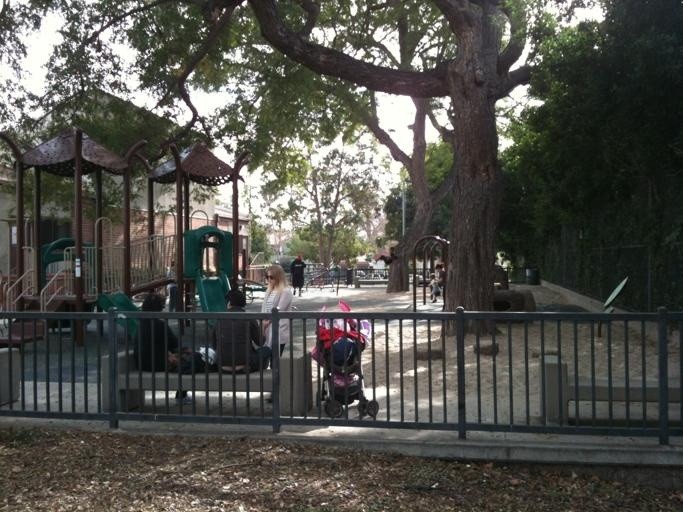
428, 273, 438, 303
327, 262, 341, 286
345, 263, 353, 287
259, 265, 293, 402
167, 261, 177, 311
290, 256, 306, 296
427, 264, 447, 299
211, 291, 271, 374
134, 292, 192, 405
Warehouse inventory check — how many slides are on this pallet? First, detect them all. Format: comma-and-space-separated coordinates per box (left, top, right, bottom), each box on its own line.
196, 270, 233, 326
98, 292, 177, 349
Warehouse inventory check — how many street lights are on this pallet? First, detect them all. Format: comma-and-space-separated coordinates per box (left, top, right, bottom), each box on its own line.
387, 126, 406, 245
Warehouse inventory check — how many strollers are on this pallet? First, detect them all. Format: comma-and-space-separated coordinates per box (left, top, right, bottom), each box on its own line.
312, 300, 384, 423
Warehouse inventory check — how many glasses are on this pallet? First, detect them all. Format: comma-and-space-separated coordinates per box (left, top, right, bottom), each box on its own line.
265, 275, 273, 280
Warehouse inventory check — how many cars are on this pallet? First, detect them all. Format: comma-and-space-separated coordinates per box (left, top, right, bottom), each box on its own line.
307, 267, 347, 286
354, 269, 430, 286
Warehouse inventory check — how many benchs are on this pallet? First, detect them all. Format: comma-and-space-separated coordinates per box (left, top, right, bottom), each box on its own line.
354, 276, 388, 287
540, 355, 683, 426
0, 347, 22, 406
99, 350, 313, 416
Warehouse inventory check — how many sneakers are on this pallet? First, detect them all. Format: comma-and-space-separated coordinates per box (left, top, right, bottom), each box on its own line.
176, 396, 194, 405
